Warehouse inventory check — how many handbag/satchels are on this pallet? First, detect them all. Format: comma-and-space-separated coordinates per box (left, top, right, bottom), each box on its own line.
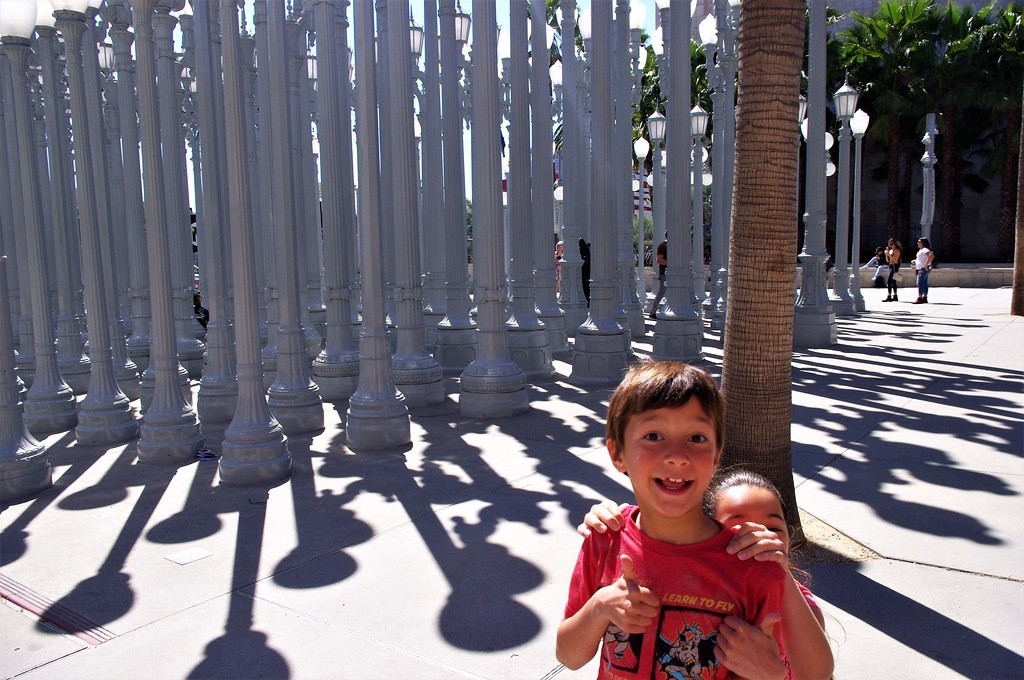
893, 273, 903, 281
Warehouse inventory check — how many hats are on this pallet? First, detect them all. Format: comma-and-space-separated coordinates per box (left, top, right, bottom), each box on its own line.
875, 247, 883, 253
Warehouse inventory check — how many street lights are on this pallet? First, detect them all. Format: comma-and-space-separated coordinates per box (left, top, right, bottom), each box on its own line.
847, 108, 869, 312
648, 98, 667, 294
0, 0, 140, 511
827, 73, 860, 316
553, 185, 563, 241
690, 92, 708, 305
796, 91, 809, 215
634, 136, 650, 308
502, 191, 509, 275
549, 59, 562, 184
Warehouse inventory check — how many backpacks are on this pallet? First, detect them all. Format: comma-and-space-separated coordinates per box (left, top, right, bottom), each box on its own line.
876, 276, 884, 288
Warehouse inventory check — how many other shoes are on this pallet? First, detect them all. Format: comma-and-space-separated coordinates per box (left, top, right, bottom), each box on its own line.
882, 296, 892, 302
913, 297, 923, 304
922, 297, 927, 303
649, 314, 657, 321
892, 295, 898, 301
872, 277, 877, 281
859, 266, 867, 271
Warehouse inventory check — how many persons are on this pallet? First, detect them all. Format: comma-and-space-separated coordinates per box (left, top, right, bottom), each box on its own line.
881, 238, 902, 304
861, 247, 889, 282
194, 293, 209, 342
911, 237, 933, 304
577, 470, 846, 680
579, 238, 591, 307
554, 233, 563, 292
556, 360, 794, 680
649, 229, 667, 319
